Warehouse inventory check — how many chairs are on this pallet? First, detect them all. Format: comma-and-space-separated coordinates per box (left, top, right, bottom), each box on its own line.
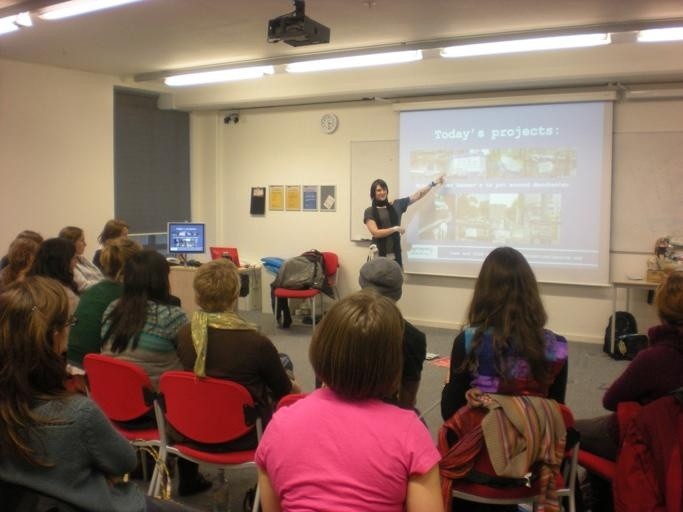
142, 367, 263, 512
446, 402, 580, 512
272, 251, 342, 331
276, 392, 311, 410
82, 351, 178, 499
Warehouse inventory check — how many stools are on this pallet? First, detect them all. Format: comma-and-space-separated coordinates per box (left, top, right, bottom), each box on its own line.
568, 439, 616, 511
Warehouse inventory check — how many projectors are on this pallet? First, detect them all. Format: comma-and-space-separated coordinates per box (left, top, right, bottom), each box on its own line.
267, 16, 330, 47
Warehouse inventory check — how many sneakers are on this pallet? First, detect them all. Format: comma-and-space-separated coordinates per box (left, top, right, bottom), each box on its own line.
176, 472, 214, 497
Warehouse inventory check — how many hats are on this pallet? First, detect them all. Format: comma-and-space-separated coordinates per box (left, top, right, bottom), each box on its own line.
359, 257, 403, 302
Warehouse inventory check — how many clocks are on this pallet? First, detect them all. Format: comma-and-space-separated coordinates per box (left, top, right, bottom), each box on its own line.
319, 110, 338, 133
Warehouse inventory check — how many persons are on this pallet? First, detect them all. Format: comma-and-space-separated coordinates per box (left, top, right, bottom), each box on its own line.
100, 250, 207, 496
442, 249, 567, 512
316, 259, 426, 409
3, 221, 144, 385
576, 271, 683, 511
172, 259, 302, 452
363, 175, 444, 274
2, 276, 180, 508
254, 289, 443, 512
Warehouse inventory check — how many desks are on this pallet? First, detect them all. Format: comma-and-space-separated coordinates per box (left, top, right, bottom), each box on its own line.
166, 263, 262, 315
607, 276, 661, 354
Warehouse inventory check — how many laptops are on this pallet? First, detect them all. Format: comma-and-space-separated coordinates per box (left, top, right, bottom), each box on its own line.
210, 247, 244, 268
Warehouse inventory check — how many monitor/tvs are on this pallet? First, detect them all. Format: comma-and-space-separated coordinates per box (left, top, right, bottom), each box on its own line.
167, 222, 206, 266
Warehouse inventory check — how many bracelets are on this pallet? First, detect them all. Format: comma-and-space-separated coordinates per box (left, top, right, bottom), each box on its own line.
431, 181, 436, 187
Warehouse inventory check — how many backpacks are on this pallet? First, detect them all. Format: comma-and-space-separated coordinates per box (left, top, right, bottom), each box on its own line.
277, 248, 325, 291
